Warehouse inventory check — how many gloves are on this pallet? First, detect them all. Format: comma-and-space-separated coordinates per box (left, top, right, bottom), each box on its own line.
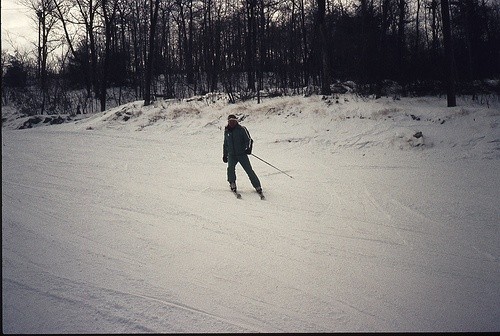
246, 149, 252, 154
222, 155, 228, 163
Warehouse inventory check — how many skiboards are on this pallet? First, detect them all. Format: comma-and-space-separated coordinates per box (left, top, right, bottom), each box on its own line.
230, 187, 265, 201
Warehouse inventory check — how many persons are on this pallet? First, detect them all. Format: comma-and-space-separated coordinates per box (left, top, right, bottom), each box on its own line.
222, 115, 266, 200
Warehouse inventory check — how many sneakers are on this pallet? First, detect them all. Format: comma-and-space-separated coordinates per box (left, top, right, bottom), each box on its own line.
255, 186, 265, 200
231, 187, 241, 198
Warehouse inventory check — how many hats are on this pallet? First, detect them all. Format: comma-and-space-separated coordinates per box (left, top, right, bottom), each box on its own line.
228, 114, 239, 122
